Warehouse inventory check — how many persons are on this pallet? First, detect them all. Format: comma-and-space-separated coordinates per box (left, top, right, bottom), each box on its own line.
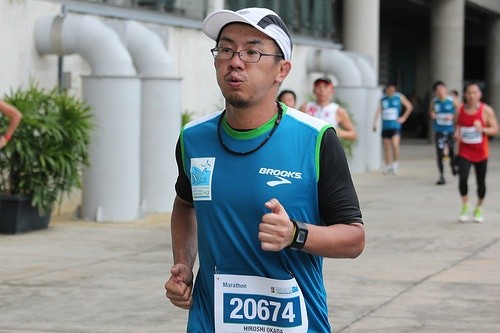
276, 89, 297, 114
0, 96, 24, 149
164, 8, 364, 333
373, 82, 414, 175
428, 80, 500, 224
297, 77, 358, 142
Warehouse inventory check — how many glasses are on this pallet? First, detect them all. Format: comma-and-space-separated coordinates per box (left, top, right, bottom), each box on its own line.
210, 47, 283, 63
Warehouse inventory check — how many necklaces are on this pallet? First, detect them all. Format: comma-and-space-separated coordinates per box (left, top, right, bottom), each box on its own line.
217, 99, 284, 156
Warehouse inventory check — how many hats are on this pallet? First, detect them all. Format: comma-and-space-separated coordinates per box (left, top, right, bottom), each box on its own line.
314, 77, 333, 88
202, 7, 293, 60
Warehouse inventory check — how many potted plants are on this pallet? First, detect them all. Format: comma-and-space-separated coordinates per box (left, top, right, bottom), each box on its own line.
0, 75, 103, 235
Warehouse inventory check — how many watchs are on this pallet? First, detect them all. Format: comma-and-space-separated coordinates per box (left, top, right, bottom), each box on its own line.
285, 217, 309, 251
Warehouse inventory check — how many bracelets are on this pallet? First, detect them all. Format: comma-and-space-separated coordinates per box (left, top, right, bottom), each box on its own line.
482, 127, 488, 136
3, 131, 11, 142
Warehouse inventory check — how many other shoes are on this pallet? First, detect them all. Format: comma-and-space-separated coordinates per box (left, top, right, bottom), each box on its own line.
451, 163, 456, 175
436, 177, 445, 185
384, 163, 397, 175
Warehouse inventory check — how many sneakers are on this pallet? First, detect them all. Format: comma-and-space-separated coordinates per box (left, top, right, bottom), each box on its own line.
473, 207, 483, 222
458, 206, 468, 222
